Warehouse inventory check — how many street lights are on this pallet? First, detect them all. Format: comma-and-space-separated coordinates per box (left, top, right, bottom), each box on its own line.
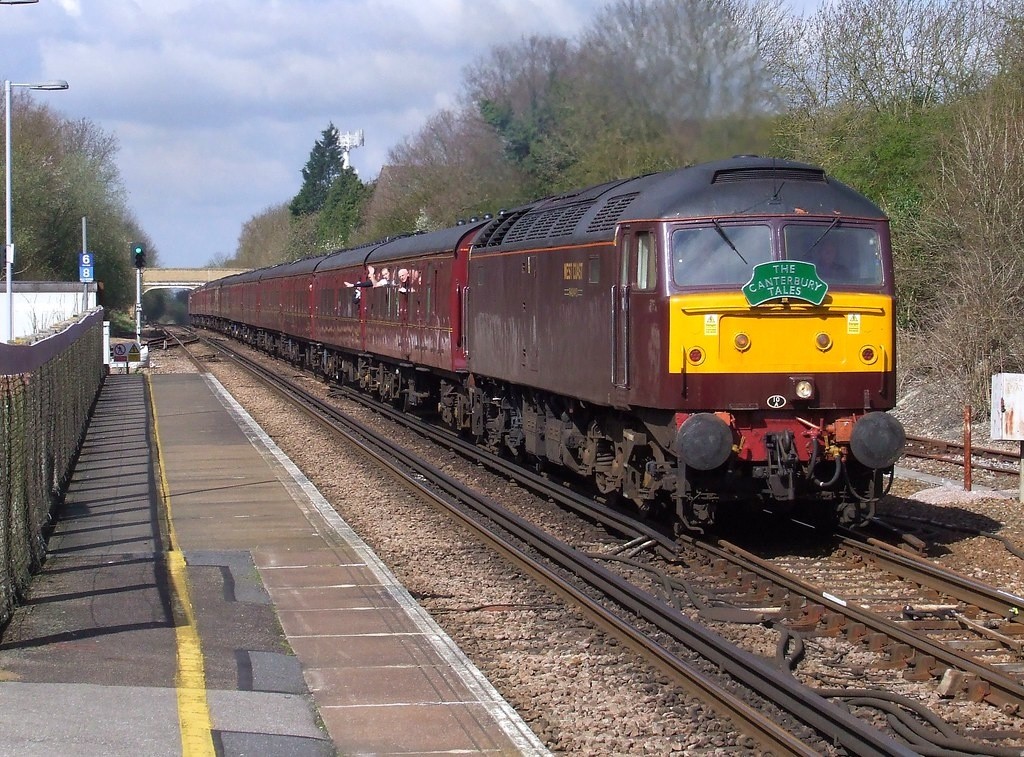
7, 79, 70, 342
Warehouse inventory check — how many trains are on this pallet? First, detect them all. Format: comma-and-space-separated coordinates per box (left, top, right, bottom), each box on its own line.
191, 157, 909, 536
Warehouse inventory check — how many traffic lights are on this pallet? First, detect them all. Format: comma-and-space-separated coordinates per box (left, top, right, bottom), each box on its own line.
130, 242, 148, 268
